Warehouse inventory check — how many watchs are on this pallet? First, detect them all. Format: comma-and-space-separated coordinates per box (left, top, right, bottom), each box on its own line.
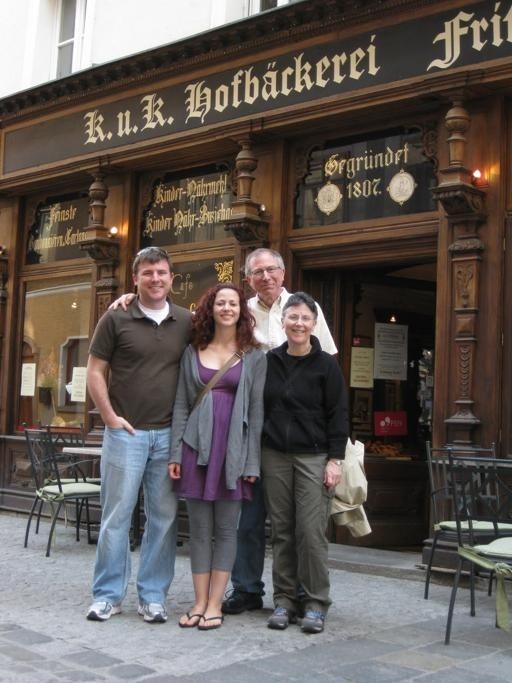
329, 457, 344, 466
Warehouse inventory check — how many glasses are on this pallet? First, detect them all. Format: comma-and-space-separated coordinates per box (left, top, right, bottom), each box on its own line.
136, 246, 169, 256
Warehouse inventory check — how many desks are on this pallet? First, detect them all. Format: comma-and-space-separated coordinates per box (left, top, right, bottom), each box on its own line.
58, 442, 185, 553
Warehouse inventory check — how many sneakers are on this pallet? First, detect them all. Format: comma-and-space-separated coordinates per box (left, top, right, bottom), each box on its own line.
301, 609, 325, 633
137, 602, 168, 623
222, 590, 263, 614
268, 605, 297, 629
86, 602, 122, 621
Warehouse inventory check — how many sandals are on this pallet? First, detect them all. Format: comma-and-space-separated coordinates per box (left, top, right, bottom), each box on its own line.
197, 613, 224, 630
180, 610, 203, 627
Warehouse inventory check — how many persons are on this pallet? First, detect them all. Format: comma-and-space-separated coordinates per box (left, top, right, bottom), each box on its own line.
168, 281, 268, 630
261, 290, 349, 632
86, 246, 200, 624
107, 246, 340, 616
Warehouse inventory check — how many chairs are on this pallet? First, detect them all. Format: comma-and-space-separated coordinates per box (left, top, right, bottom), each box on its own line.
20, 419, 103, 559
34, 421, 103, 543
421, 436, 510, 604
443, 444, 511, 649
